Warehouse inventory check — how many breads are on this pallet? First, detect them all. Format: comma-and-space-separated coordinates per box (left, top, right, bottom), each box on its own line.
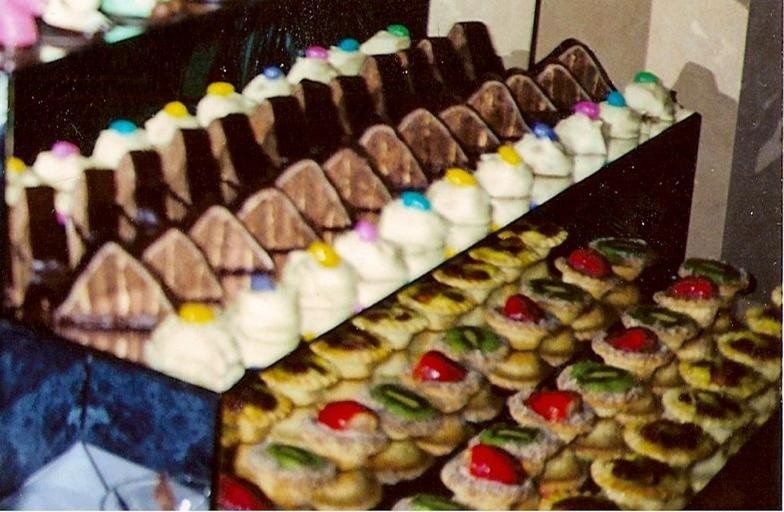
214, 218, 782, 512
0, 0, 220, 74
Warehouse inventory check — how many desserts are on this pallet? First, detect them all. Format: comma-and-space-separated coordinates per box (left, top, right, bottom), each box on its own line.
1, 21, 696, 393
